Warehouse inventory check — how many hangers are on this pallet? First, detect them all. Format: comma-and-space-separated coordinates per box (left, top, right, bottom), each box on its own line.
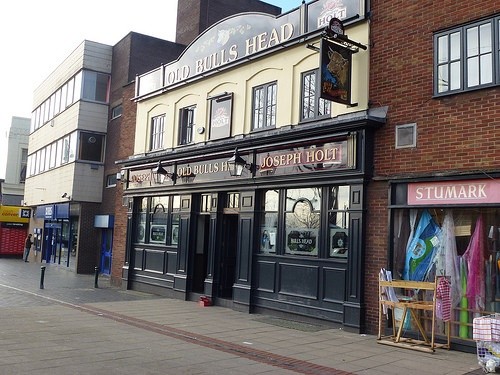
382, 267, 387, 275
440, 269, 451, 284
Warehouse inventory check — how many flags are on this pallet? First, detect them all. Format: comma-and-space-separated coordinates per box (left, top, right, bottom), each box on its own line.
402, 208, 446, 291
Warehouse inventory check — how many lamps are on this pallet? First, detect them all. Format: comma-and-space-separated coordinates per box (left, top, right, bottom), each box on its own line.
227, 145, 252, 178
150, 159, 176, 185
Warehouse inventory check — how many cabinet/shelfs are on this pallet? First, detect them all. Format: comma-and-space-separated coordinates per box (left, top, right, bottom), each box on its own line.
377, 272, 450, 353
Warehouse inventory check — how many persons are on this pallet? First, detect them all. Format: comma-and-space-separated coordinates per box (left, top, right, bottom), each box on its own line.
24, 234, 33, 262
